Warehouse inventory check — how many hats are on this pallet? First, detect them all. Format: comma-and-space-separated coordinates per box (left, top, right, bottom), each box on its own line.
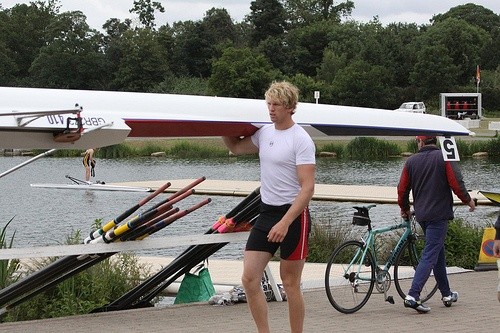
416, 135, 436, 139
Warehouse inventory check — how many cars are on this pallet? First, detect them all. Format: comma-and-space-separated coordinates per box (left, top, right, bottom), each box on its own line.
395, 102, 426, 114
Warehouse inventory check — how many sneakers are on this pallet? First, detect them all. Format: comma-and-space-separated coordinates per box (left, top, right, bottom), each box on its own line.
404, 299, 431, 313
443, 292, 458, 307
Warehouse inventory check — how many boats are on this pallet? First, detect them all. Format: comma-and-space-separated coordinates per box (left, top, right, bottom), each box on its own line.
0, 85, 477, 150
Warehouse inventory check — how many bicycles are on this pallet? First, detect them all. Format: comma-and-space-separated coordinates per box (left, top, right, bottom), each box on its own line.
324, 203, 440, 315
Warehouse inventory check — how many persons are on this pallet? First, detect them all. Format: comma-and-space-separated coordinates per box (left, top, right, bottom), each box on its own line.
222, 81, 316, 333
83, 148, 96, 181
396, 135, 475, 312
